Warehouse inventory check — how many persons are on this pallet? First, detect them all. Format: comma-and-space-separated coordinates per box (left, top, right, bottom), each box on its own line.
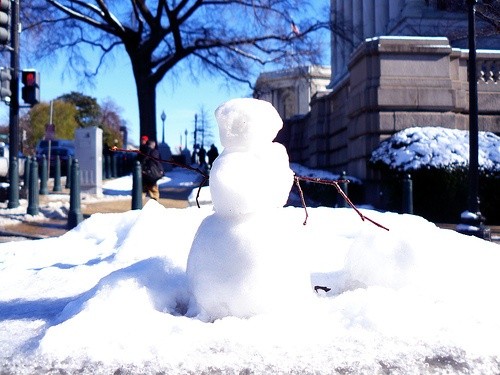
140, 136, 160, 203
206, 144, 219, 169
197, 144, 206, 166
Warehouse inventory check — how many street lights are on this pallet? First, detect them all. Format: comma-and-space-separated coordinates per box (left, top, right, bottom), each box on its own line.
160, 110, 166, 144
184, 129, 188, 149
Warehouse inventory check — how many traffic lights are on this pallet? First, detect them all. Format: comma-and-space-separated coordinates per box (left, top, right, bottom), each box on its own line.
0, 67, 12, 100
21, 71, 40, 105
0, 0, 11, 46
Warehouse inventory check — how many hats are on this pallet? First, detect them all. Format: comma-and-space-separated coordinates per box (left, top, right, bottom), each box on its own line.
142, 135, 149, 142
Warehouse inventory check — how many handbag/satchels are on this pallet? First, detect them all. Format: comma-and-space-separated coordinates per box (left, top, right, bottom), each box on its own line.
144, 161, 166, 183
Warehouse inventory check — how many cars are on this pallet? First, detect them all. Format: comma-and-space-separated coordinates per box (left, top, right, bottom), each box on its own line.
0, 142, 32, 181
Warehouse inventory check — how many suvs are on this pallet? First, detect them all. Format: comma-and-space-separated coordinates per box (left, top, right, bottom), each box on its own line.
35, 139, 74, 178
